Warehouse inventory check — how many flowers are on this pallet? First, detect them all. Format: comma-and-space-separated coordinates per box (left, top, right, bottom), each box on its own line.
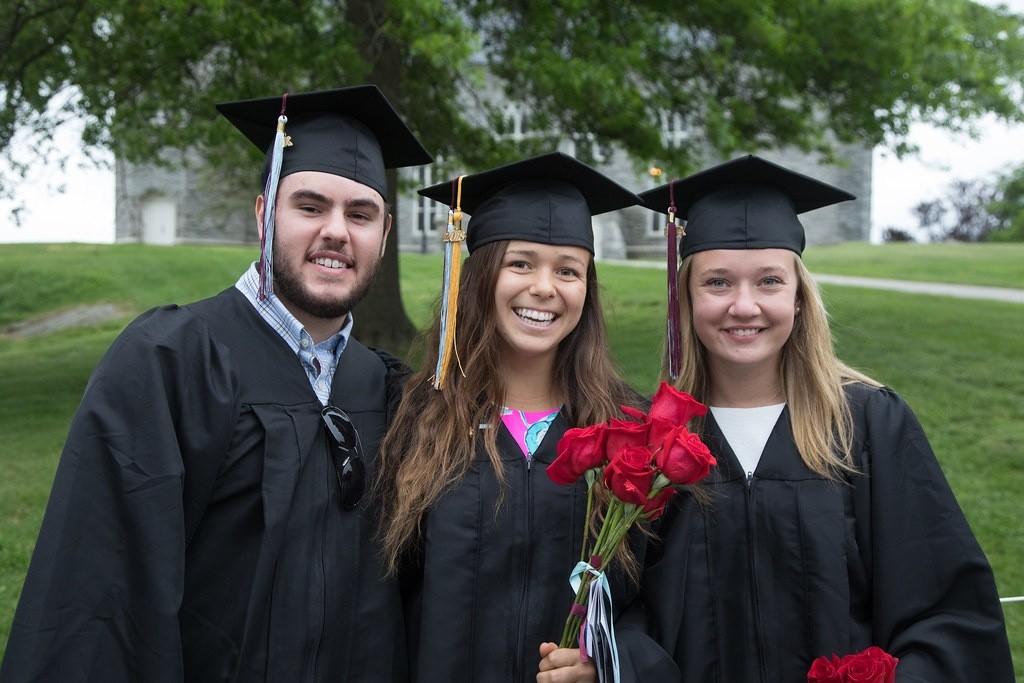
547, 381, 717, 648
808, 646, 899, 683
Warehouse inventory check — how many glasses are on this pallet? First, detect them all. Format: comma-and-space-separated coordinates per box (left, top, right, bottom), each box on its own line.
321, 405, 368, 510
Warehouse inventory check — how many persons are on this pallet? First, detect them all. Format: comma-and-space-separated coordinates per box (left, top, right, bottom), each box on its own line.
634, 151, 1023, 683
1, 84, 404, 682
418, 149, 655, 683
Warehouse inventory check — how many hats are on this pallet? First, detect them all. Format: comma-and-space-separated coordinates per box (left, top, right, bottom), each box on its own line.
637, 153, 857, 380
417, 151, 644, 390
215, 84, 434, 302
